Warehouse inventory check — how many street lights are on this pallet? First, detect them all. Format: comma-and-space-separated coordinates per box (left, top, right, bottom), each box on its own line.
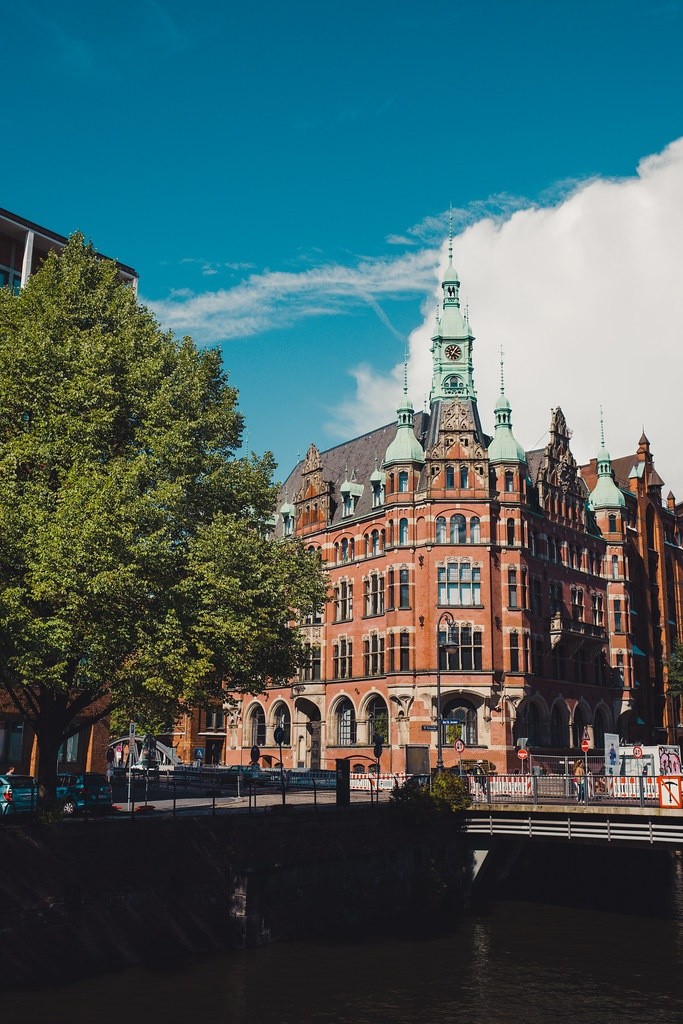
436, 612, 460, 775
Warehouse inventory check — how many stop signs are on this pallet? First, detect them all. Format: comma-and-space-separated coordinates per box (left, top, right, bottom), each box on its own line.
581, 739, 590, 752
517, 749, 529, 760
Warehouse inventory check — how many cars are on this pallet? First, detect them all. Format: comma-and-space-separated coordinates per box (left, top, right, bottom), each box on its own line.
271, 769, 337, 789
218, 765, 272, 787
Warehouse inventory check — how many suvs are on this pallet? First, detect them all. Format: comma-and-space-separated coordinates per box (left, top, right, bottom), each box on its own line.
53, 771, 112, 818
0, 775, 51, 817
